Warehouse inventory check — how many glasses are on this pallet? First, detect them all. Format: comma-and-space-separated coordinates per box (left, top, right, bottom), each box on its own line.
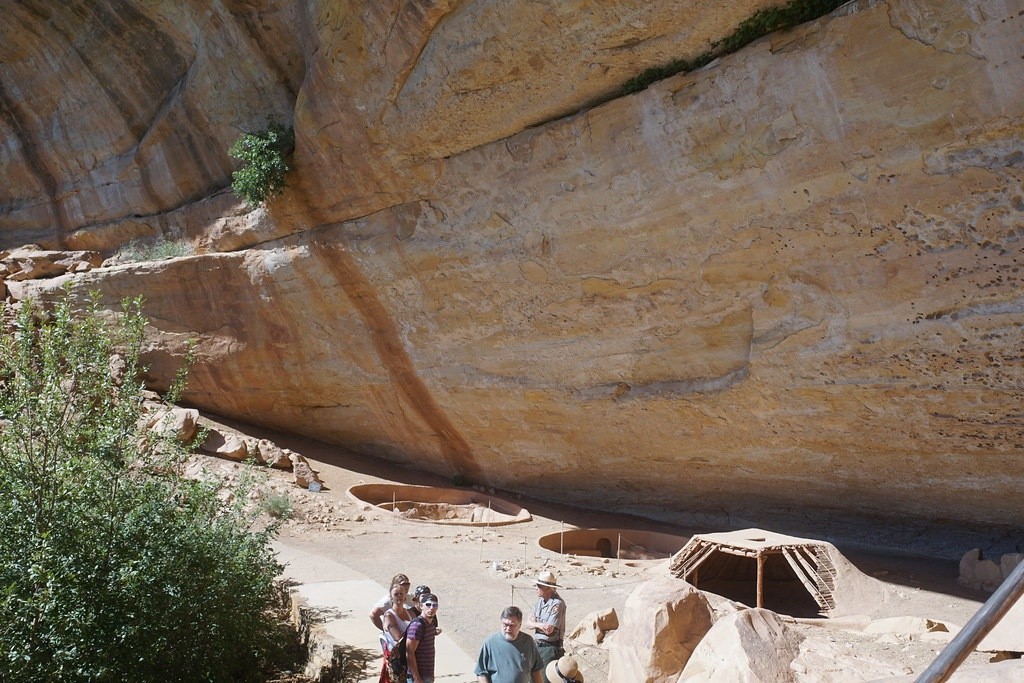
422, 603, 438, 607
394, 593, 404, 596
400, 583, 410, 586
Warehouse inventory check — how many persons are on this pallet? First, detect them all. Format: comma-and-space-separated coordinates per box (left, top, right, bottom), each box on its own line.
474, 606, 545, 683
526, 572, 566, 683
369, 574, 414, 633
545, 655, 584, 683
379, 583, 417, 683
409, 585, 442, 635
406, 594, 438, 683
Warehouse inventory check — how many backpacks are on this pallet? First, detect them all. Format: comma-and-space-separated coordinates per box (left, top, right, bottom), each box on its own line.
386, 619, 426, 682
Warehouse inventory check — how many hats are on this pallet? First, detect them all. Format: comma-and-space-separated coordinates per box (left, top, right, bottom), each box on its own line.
412, 586, 430, 601
529, 571, 562, 589
545, 655, 584, 683
